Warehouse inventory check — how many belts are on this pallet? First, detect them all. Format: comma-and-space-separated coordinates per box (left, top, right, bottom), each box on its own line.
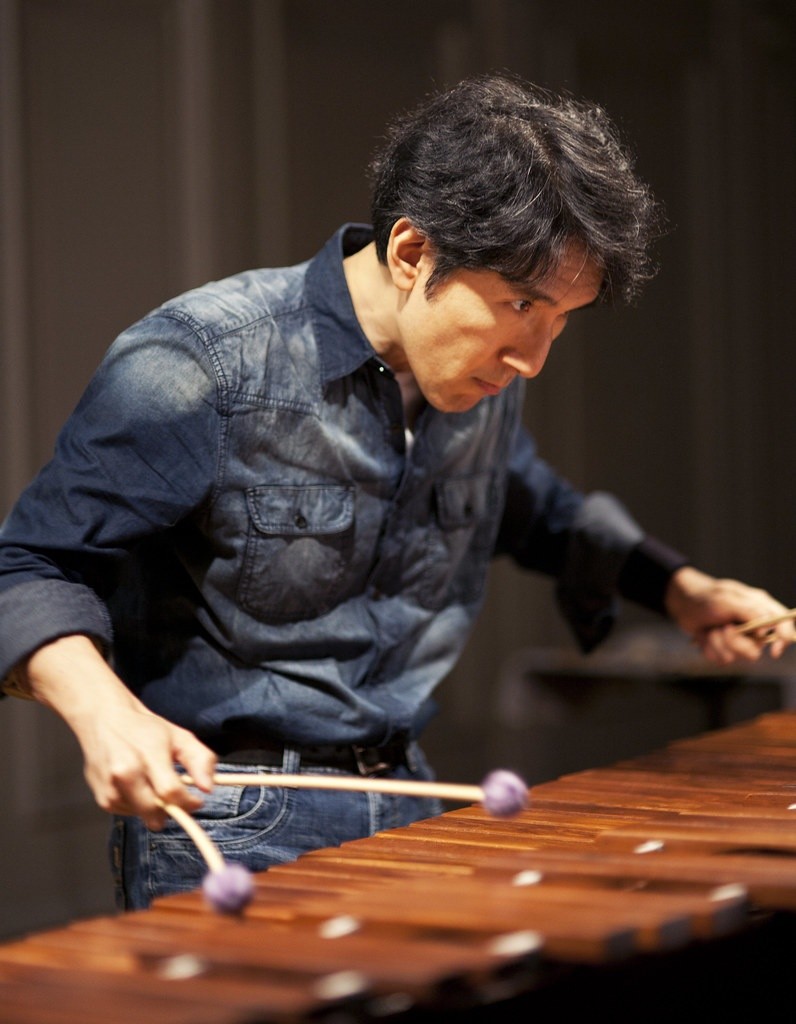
209, 732, 416, 777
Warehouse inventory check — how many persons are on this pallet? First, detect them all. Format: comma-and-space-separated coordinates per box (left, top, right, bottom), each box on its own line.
0, 74, 795, 915
